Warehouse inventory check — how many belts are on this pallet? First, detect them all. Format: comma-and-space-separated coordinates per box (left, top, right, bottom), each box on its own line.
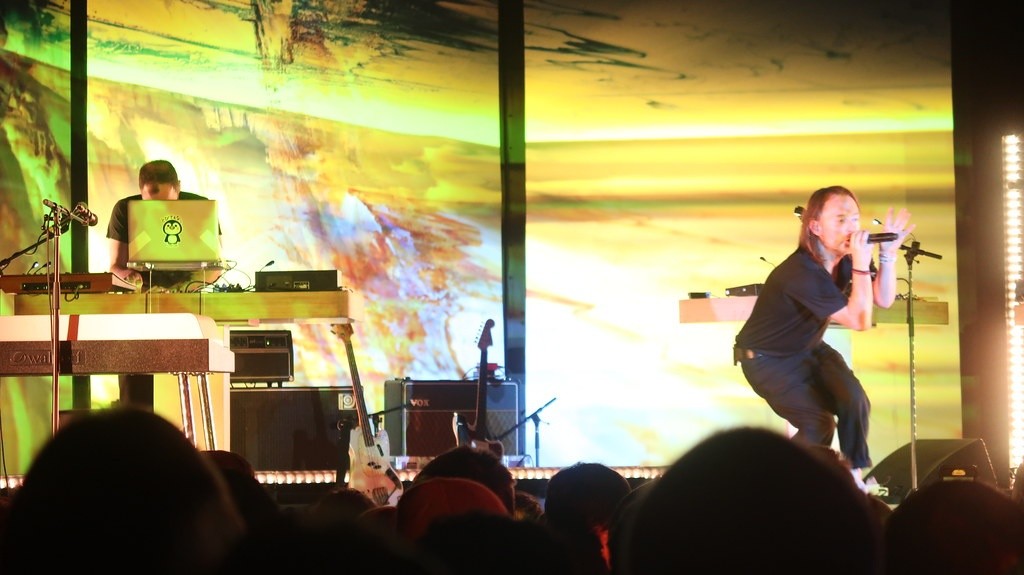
742, 349, 762, 359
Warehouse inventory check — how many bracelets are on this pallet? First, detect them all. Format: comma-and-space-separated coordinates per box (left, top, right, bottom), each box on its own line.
879, 253, 897, 263
851, 269, 871, 275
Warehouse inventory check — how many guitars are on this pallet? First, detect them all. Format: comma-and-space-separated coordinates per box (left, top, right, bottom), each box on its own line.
450, 318, 505, 464
330, 318, 403, 508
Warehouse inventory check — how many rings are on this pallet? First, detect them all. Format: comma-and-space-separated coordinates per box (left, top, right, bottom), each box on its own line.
161, 288, 163, 293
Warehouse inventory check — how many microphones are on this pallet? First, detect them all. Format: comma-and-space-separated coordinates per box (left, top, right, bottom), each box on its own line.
75, 205, 98, 226
260, 261, 274, 272
867, 233, 902, 245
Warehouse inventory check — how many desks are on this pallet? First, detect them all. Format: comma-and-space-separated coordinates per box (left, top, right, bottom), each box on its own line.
0, 337, 236, 411
0, 293, 350, 411
678, 296, 949, 475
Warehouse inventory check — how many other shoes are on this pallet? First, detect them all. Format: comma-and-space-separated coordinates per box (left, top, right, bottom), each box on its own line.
850, 465, 890, 498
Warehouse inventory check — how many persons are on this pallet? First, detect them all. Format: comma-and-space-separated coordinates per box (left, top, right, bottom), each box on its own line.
734, 185, 917, 494
1, 410, 1024, 575
106, 160, 222, 413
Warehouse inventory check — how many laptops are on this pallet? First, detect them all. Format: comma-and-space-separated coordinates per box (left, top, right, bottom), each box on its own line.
127, 200, 223, 271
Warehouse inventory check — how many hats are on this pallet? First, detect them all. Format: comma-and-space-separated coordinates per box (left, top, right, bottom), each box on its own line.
305, 488, 397, 542
394, 477, 511, 556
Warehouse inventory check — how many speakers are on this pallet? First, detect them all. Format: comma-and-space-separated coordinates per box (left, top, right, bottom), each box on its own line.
862, 438, 998, 505
229, 386, 361, 472
384, 379, 526, 470
